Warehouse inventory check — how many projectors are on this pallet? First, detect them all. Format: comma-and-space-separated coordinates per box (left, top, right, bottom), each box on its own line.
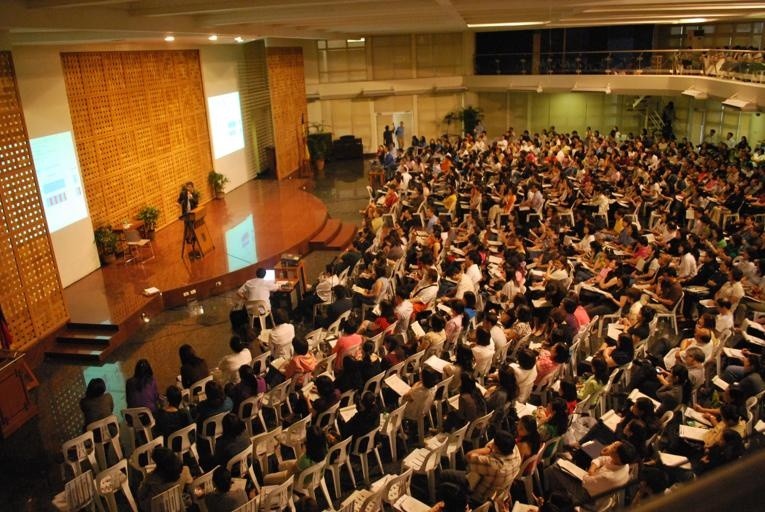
280, 251, 303, 265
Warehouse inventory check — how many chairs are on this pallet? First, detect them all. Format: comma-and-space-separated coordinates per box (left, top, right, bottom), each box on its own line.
51, 134, 765, 512
653, 55, 765, 85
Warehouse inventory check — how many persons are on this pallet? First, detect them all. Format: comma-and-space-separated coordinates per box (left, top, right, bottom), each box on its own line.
52, 120, 764, 509
489, 43, 765, 77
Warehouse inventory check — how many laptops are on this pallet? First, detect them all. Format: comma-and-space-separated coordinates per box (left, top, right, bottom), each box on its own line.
263, 269, 276, 285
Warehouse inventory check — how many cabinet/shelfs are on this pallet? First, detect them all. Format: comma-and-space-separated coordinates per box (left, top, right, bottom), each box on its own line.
0, 352, 40, 439
